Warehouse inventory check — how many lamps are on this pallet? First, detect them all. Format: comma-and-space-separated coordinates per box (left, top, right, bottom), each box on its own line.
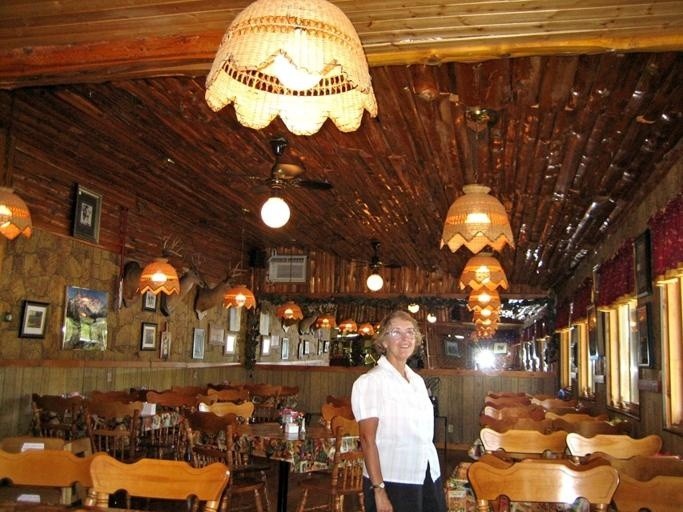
465, 291, 502, 342
225, 208, 259, 310
0, 185, 33, 240
365, 273, 385, 292
404, 300, 420, 315
137, 154, 182, 298
197, 0, 382, 139
423, 103, 517, 289
315, 309, 375, 338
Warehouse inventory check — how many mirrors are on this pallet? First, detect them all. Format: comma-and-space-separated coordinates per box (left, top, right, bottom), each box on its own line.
253, 291, 560, 376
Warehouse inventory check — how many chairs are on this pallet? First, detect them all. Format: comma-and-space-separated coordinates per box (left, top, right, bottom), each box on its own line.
438, 383, 682, 510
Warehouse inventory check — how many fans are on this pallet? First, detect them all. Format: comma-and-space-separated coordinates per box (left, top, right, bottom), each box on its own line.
335, 238, 404, 273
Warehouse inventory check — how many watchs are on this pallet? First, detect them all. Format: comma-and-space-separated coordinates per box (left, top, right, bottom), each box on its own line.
371, 483, 384, 489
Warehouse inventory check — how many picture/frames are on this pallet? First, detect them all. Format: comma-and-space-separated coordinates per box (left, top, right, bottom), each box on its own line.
493, 342, 507, 354
254, 328, 331, 362
442, 338, 459, 357
71, 184, 103, 244
130, 291, 241, 361
588, 227, 656, 381
17, 299, 50, 339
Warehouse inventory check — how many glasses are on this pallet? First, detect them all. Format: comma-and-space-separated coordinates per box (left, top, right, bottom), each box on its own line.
385, 331, 415, 337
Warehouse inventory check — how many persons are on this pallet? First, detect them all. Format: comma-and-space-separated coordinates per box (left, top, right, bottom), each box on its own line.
349, 309, 448, 512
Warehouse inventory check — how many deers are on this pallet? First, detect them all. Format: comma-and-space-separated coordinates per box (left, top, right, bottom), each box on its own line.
282, 300, 317, 334
120, 234, 186, 309
160, 251, 208, 315
310, 304, 338, 335
299, 303, 332, 335
194, 257, 251, 321
